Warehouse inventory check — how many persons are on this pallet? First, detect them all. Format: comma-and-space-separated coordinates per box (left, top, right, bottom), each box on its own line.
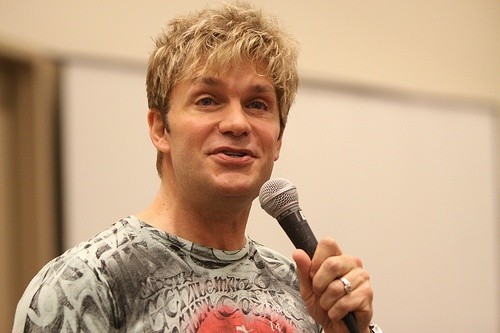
11, 0, 385, 332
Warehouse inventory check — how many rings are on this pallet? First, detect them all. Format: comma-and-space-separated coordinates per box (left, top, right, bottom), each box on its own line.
339, 275, 352, 293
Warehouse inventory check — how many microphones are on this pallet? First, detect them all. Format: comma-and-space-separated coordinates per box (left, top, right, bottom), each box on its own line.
259, 178, 362, 333
